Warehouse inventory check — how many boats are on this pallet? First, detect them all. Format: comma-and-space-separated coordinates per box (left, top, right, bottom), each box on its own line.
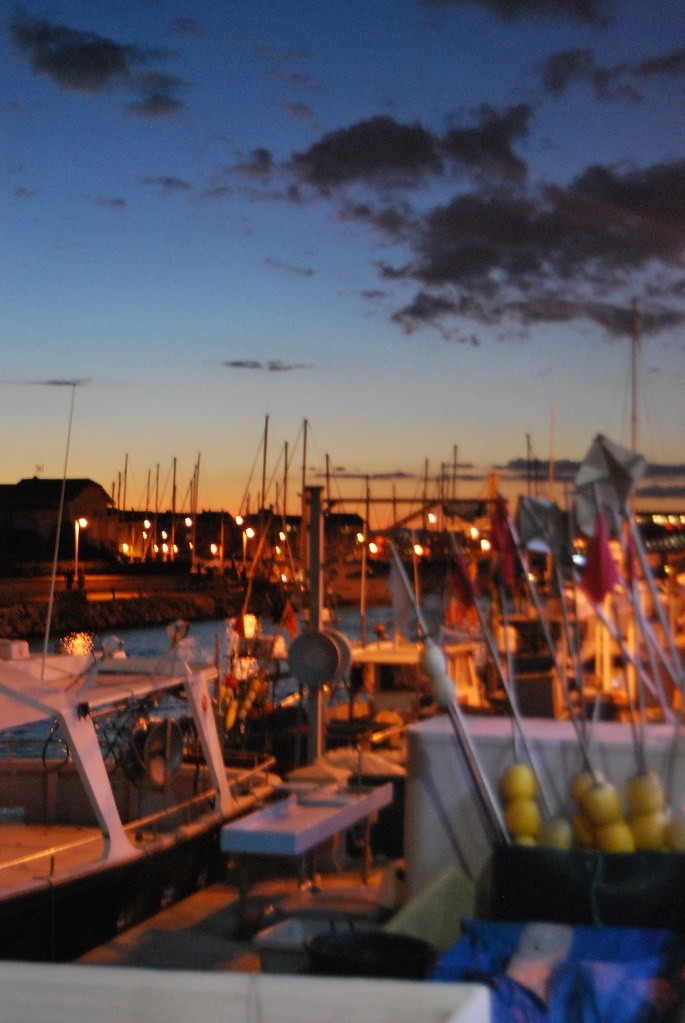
0, 636, 493, 960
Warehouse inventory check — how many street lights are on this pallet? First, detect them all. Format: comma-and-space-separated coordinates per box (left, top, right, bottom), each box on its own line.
356, 522, 424, 649
236, 516, 255, 565
73, 518, 88, 580
